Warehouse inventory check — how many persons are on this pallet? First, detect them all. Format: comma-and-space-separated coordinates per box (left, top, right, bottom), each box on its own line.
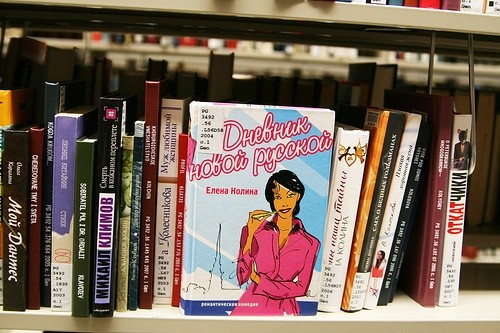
375, 251, 386, 270
455, 129, 470, 171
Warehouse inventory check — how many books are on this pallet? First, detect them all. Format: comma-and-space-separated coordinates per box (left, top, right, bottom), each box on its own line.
0, 0, 500, 317
